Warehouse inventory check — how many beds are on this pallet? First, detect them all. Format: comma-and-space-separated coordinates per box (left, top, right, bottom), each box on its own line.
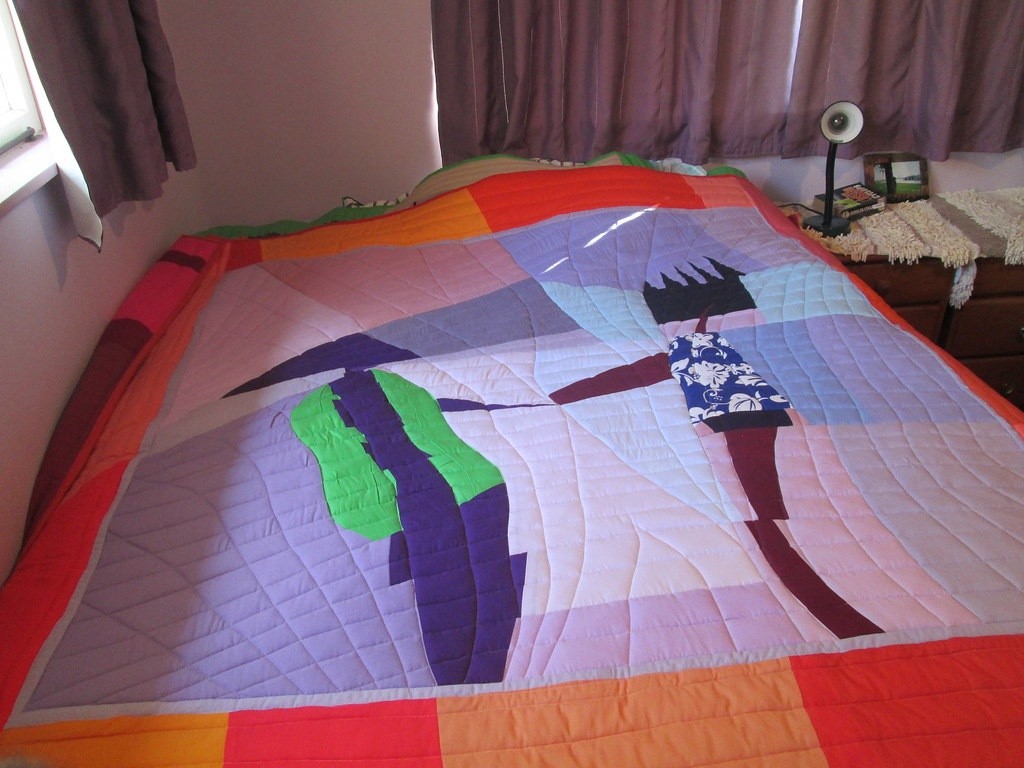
0, 146, 1024, 768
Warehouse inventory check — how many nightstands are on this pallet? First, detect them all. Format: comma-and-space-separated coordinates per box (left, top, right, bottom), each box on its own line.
830, 251, 1023, 417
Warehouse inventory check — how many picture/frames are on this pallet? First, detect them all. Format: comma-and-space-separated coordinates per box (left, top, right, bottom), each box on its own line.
863, 151, 931, 202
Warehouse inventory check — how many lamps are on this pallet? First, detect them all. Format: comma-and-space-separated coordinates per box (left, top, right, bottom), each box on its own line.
802, 101, 864, 238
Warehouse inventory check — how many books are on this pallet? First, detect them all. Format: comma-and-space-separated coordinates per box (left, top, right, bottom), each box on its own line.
814, 181, 887, 220
